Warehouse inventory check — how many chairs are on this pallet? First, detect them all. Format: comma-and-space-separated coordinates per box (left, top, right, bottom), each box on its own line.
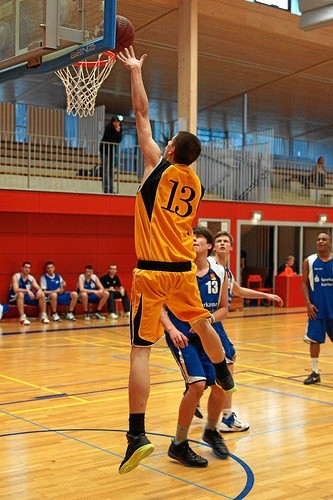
246, 275, 272, 306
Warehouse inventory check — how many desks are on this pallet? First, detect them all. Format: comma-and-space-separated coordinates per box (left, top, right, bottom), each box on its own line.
275, 276, 306, 308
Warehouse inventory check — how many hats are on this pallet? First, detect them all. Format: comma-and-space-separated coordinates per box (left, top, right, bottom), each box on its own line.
110, 115, 120, 122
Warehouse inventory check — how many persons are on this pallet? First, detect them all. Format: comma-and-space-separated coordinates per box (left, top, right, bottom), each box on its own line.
279, 256, 296, 274
303, 232, 333, 385
194, 231, 283, 432
312, 156, 326, 186
9, 262, 50, 324
241, 249, 246, 275
99, 116, 123, 193
76, 265, 110, 319
100, 264, 130, 319
117, 46, 237, 475
40, 261, 79, 320
161, 227, 229, 468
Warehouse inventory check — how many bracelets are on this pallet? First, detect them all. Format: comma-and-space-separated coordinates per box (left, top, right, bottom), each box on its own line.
209, 314, 215, 324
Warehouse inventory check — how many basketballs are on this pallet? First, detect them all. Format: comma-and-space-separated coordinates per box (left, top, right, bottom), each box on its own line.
94, 14, 136, 55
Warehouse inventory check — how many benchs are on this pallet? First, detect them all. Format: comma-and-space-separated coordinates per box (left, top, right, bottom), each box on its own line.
0, 140, 141, 183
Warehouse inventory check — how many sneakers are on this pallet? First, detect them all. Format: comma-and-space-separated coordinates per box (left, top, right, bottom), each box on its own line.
183, 389, 203, 419
108, 313, 118, 319
123, 311, 130, 317
52, 313, 60, 321
19, 318, 30, 325
304, 371, 321, 385
84, 313, 90, 321
219, 412, 250, 433
65, 313, 74, 320
40, 316, 49, 323
119, 433, 154, 474
92, 312, 105, 319
167, 441, 208, 468
203, 426, 228, 458
215, 374, 237, 396
